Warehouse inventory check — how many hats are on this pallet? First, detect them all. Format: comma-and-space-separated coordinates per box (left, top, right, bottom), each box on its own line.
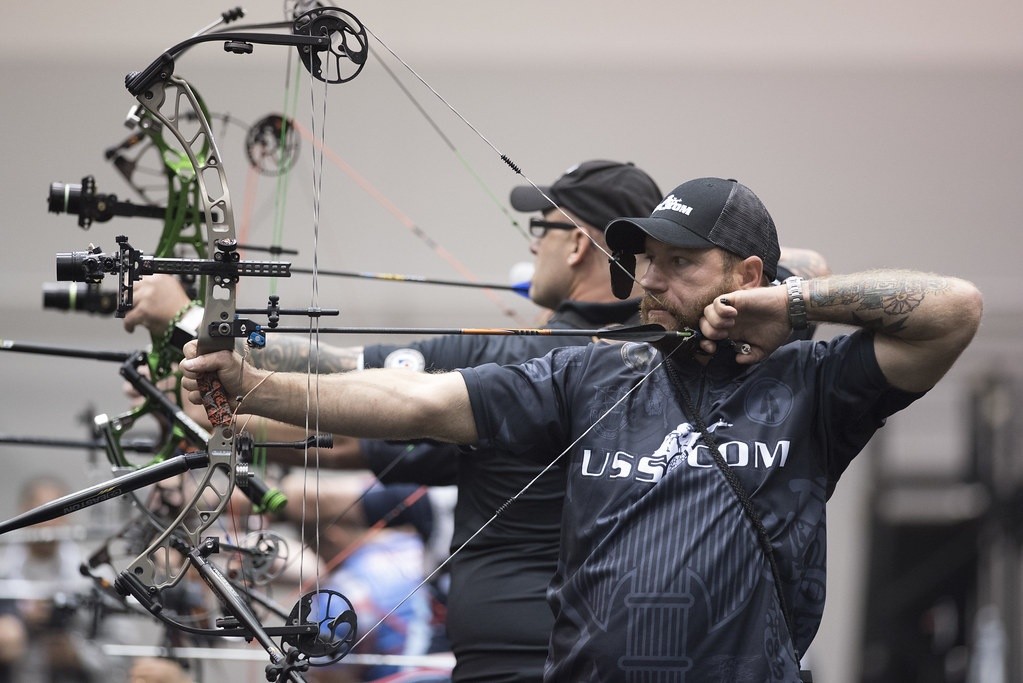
511, 159, 663, 233
605, 177, 781, 283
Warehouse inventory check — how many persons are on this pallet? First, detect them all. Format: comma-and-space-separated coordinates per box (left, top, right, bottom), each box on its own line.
0, 465, 458, 683
121, 161, 829, 683
179, 177, 983, 683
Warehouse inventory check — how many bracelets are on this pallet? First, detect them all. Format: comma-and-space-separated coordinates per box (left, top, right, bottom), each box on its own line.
783, 276, 810, 331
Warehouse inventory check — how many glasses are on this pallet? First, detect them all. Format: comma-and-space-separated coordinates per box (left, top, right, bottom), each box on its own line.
528, 217, 577, 240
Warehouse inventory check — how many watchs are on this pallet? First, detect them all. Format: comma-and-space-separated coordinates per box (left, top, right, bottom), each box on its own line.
169, 305, 205, 350
161, 300, 202, 346
